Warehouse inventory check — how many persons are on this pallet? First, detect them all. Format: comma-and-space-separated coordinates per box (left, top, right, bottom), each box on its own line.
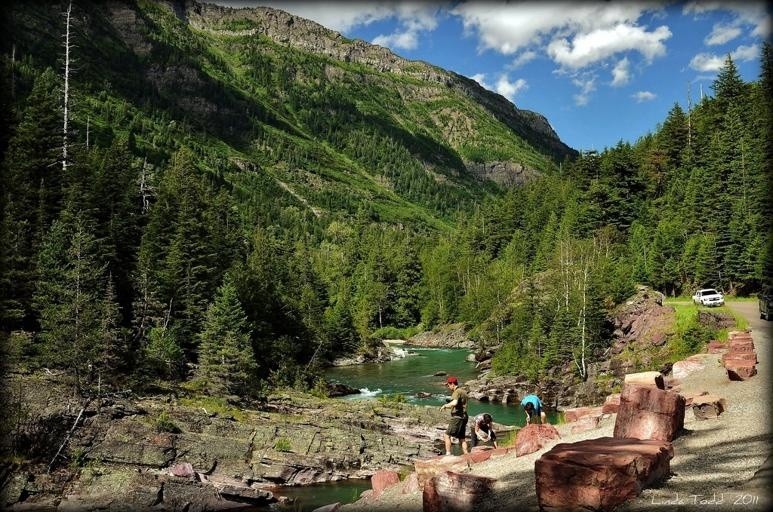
440, 377, 468, 457
470, 412, 498, 451
521, 394, 546, 426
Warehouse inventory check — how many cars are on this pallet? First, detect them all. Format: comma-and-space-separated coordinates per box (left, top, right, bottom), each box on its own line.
692, 289, 724, 308
755, 285, 773, 322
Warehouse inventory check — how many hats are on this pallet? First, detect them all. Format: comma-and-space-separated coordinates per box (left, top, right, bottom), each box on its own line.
444, 377, 457, 385
